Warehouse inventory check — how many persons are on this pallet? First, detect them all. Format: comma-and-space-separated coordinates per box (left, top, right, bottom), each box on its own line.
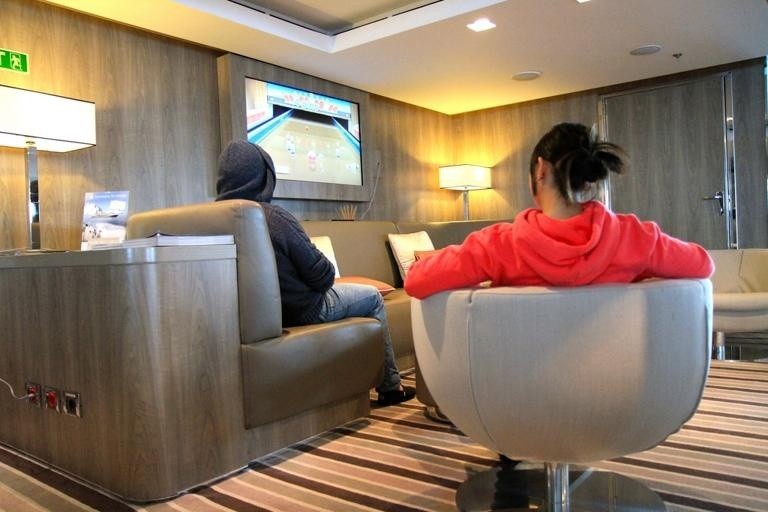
215, 138, 415, 407
404, 121, 714, 424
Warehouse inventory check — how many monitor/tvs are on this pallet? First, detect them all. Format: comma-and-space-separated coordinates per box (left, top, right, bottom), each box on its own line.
243, 75, 363, 186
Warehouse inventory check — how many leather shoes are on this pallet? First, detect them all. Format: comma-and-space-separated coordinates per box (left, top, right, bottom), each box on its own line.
378, 384, 416, 407
424, 405, 450, 423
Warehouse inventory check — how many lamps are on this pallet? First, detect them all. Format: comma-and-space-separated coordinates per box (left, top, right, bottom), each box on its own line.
0, 84, 97, 249
440, 164, 492, 220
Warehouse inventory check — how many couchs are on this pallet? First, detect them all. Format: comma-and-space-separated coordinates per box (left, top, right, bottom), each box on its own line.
705, 249, 768, 360
0, 199, 386, 505
404, 276, 715, 512
295, 220, 515, 371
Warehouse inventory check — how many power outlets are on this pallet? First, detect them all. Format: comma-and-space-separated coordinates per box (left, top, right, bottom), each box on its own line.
64, 391, 81, 418
25, 383, 41, 408
43, 385, 61, 415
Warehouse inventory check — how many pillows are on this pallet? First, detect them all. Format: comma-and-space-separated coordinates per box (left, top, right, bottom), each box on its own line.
308, 236, 340, 279
387, 231, 436, 281
333, 276, 396, 297
